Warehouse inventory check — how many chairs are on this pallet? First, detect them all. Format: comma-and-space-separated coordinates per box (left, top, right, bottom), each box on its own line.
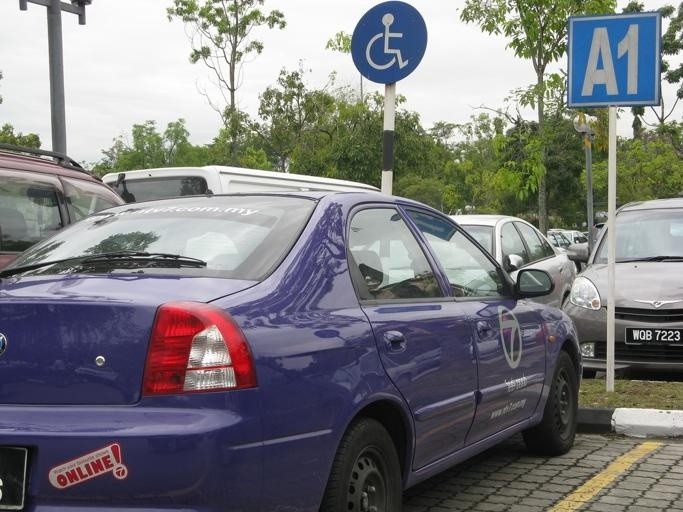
351, 248, 384, 290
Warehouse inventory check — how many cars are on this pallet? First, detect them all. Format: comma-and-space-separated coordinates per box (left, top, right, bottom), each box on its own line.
0, 189, 581, 510
541, 223, 604, 260
367, 213, 577, 308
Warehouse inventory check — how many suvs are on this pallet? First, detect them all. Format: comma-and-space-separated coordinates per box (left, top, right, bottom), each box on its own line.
0, 143, 130, 275
561, 198, 683, 374
99, 165, 381, 205
